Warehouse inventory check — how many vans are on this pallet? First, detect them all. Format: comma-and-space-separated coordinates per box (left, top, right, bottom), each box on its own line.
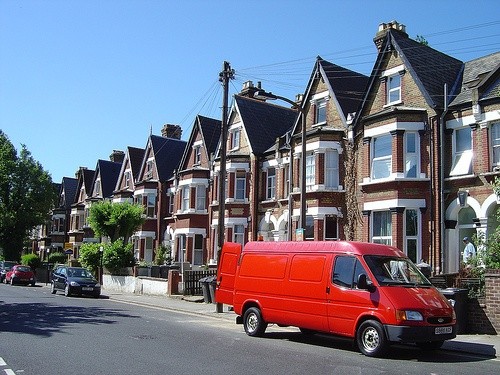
214, 241, 457, 357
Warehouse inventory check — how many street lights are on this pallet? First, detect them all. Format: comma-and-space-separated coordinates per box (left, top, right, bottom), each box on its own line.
253, 90, 306, 241
47, 248, 50, 283
100, 246, 104, 284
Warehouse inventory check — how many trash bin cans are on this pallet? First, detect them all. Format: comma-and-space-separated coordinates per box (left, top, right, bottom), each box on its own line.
440, 286, 470, 335
198, 275, 217, 304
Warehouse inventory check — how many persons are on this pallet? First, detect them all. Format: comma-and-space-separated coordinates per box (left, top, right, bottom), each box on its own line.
461, 237, 477, 266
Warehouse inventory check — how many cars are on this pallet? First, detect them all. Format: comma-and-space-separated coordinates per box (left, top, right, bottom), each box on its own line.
50, 267, 101, 298
0, 261, 20, 283
5, 265, 36, 287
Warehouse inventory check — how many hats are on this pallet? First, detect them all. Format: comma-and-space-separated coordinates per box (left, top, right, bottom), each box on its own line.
462, 237, 470, 241
